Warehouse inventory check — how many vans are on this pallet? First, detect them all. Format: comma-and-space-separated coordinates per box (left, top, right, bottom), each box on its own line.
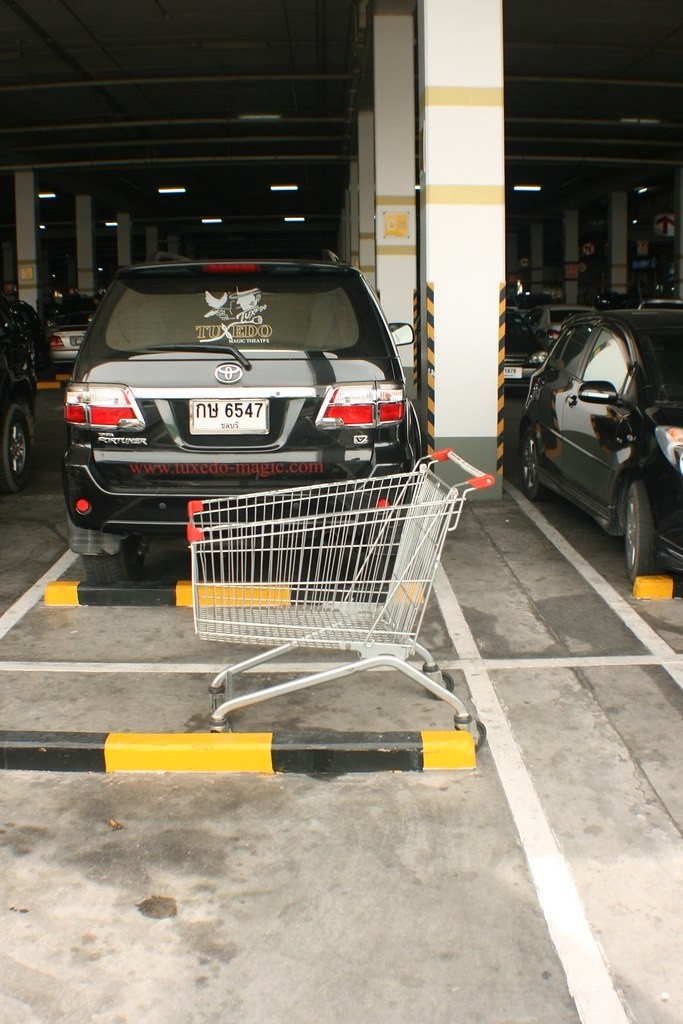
594, 298, 682, 313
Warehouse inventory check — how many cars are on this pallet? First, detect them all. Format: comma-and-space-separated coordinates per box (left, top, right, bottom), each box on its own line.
525, 304, 598, 349
46, 296, 97, 363
504, 307, 549, 396
1, 288, 42, 493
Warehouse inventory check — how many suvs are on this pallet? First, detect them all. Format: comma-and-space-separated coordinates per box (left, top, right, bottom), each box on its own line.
62, 251, 416, 579
520, 297, 683, 584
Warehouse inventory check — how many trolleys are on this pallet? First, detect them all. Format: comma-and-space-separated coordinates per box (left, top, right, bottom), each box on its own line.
190, 447, 495, 753
